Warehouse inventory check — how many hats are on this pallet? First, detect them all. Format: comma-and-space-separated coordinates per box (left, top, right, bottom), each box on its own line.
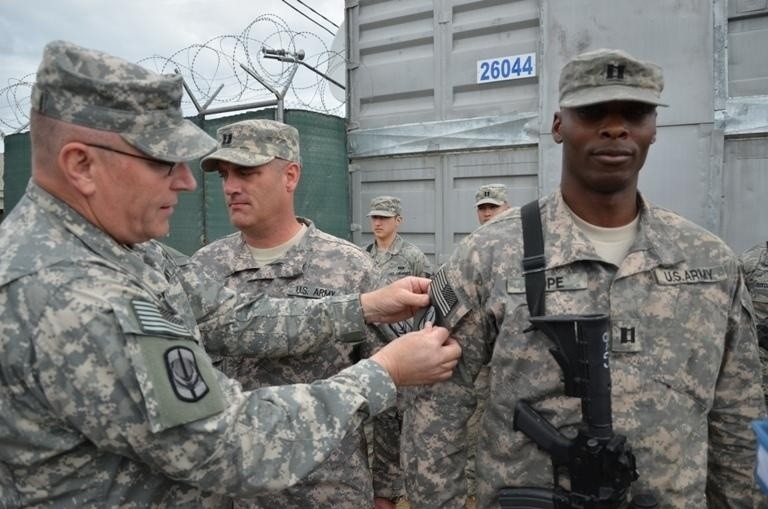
30, 40, 217, 163
201, 119, 300, 173
474, 183, 506, 207
558, 50, 670, 109
366, 197, 400, 219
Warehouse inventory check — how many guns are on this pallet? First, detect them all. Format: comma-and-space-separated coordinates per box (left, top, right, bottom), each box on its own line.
497, 312, 638, 508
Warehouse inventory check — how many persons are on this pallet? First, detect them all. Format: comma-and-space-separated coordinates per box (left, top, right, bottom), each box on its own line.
405, 47, 766, 509
359, 188, 440, 285
0, 38, 462, 509
188, 115, 402, 509
740, 238, 767, 402
472, 176, 512, 229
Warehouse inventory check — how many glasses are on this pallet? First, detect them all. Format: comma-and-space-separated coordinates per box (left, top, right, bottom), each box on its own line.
80, 141, 176, 176
